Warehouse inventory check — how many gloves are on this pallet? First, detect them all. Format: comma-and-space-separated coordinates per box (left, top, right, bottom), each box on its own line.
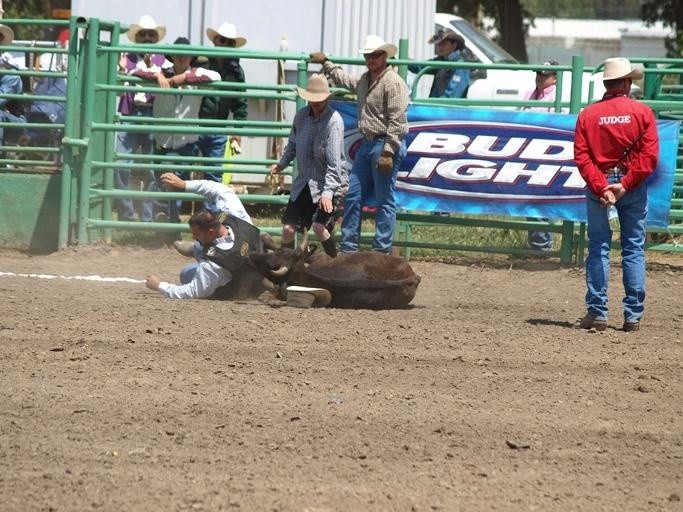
309, 52, 328, 64
375, 151, 393, 172
230, 135, 242, 157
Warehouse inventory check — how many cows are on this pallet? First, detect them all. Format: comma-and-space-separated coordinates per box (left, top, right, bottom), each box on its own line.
247, 227, 422, 311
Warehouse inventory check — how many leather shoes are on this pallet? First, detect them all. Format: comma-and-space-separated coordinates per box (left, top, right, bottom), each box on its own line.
580, 319, 607, 331
623, 321, 639, 332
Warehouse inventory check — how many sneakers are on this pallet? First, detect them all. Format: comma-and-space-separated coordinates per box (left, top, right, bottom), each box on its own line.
154, 212, 168, 237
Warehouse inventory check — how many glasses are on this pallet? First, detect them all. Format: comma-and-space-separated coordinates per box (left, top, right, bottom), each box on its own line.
218, 37, 232, 46
139, 32, 154, 37
364, 51, 384, 59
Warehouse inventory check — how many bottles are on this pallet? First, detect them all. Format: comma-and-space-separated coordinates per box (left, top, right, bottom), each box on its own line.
607, 205, 621, 231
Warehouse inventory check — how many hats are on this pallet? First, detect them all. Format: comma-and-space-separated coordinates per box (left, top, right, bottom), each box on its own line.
533, 59, 559, 75
295, 73, 341, 103
0, 24, 14, 44
164, 37, 199, 64
286, 285, 332, 308
355, 36, 397, 59
590, 58, 643, 83
206, 23, 248, 49
127, 15, 166, 44
428, 27, 464, 51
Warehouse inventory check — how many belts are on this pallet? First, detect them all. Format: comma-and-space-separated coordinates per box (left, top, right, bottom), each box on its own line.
365, 135, 384, 142
604, 167, 625, 174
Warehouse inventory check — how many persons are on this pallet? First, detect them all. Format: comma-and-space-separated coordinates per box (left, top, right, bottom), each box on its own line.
1, 24, 70, 169
144, 171, 270, 300
115, 14, 249, 243
269, 70, 350, 258
513, 58, 567, 261
572, 56, 660, 333
407, 26, 471, 99
309, 32, 410, 256
